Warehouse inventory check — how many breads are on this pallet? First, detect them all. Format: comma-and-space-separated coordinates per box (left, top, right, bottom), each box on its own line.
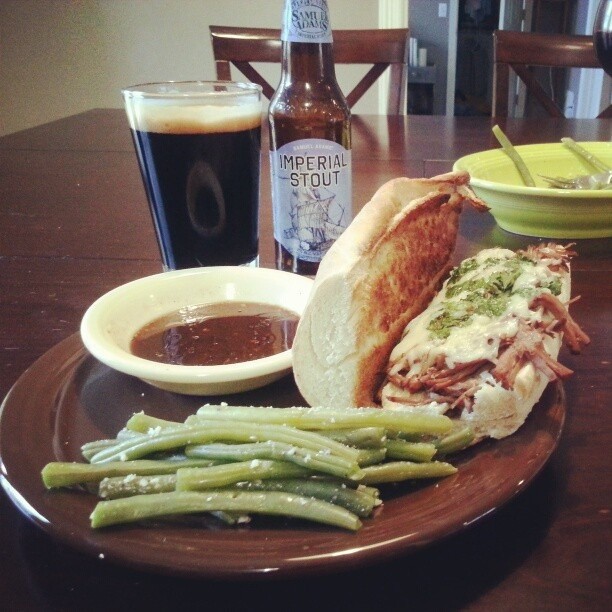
291, 170, 592, 441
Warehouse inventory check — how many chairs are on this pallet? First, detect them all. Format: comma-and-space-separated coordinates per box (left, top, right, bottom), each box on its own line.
207, 22, 410, 117
492, 28, 612, 120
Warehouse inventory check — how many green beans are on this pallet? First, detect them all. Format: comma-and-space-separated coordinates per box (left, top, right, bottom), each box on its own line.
561, 136, 611, 174
40, 403, 474, 532
490, 122, 536, 189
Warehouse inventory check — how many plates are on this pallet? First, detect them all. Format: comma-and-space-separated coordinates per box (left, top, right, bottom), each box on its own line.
1, 331, 566, 584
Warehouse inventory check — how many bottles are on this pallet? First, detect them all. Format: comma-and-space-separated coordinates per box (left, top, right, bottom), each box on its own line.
267, 0, 352, 275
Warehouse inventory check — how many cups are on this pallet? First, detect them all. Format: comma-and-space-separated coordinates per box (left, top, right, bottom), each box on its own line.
122, 80, 264, 269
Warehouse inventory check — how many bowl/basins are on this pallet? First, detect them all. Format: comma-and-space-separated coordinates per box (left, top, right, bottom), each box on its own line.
80, 265, 319, 394
451, 140, 612, 241
